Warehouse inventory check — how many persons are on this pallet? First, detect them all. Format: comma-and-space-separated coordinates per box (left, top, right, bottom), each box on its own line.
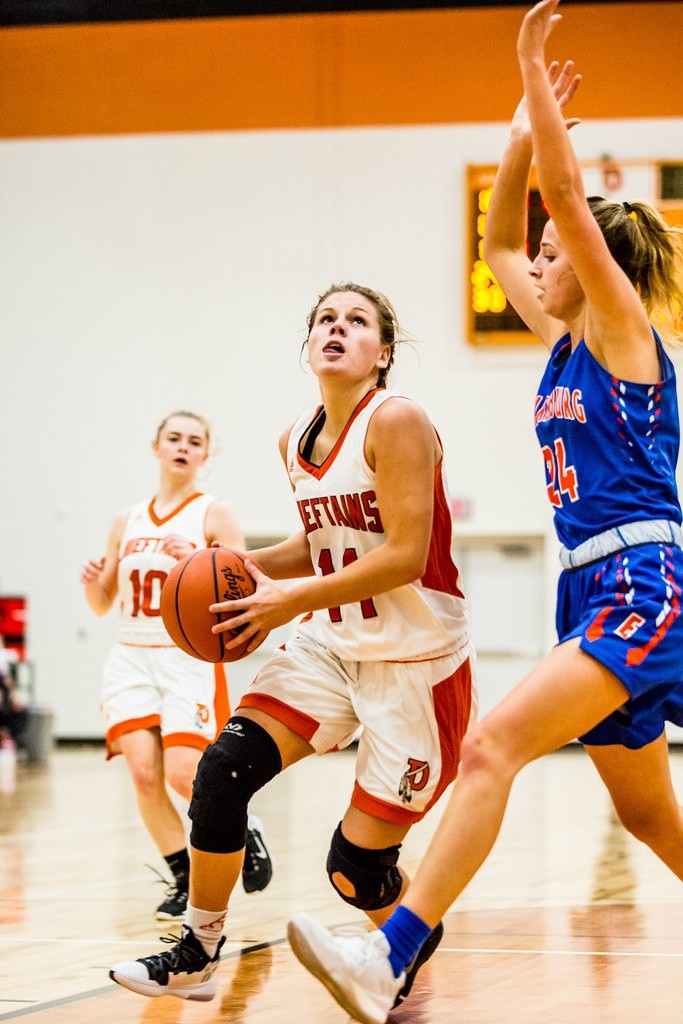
287, 0, 683, 1024
80, 408, 275, 922
110, 281, 479, 1003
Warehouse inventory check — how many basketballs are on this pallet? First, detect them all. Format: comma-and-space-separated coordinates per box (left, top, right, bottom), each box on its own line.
159, 547, 263, 664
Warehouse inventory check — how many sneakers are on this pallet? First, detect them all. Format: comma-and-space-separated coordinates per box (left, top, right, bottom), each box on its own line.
109, 923, 227, 1002
389, 921, 443, 1012
242, 814, 273, 895
144, 864, 189, 920
287, 912, 407, 1024
